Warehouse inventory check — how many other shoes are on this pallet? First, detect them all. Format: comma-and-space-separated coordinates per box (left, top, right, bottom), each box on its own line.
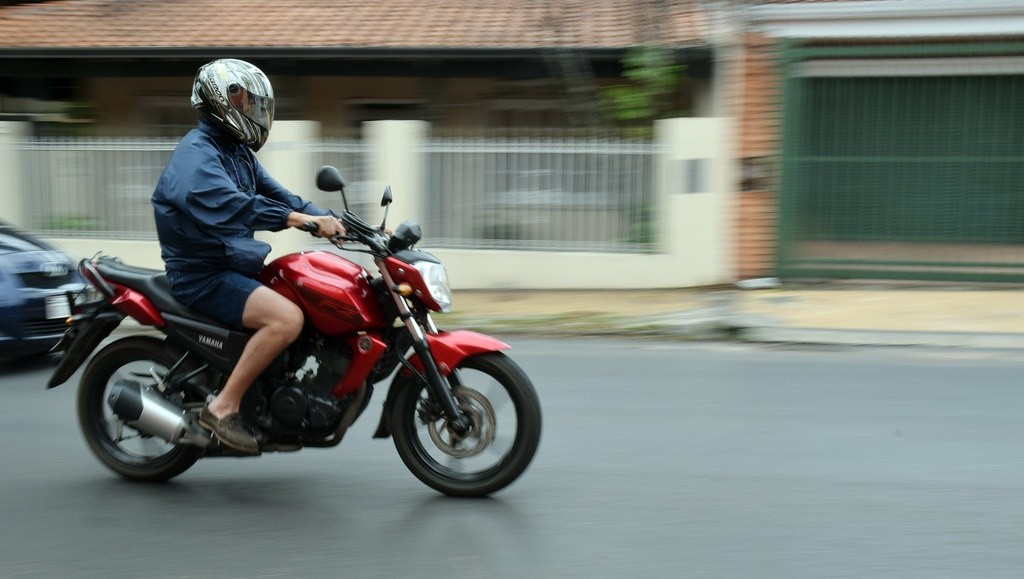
199, 403, 257, 453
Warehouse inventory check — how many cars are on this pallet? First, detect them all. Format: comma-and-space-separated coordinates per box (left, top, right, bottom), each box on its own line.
0, 219, 104, 366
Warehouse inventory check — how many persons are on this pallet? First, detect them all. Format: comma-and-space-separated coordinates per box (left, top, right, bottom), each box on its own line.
150, 60, 393, 454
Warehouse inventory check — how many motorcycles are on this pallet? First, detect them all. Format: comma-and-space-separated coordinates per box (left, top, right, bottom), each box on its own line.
45, 165, 544, 498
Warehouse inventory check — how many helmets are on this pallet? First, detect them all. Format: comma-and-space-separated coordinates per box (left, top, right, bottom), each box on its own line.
191, 58, 274, 153
